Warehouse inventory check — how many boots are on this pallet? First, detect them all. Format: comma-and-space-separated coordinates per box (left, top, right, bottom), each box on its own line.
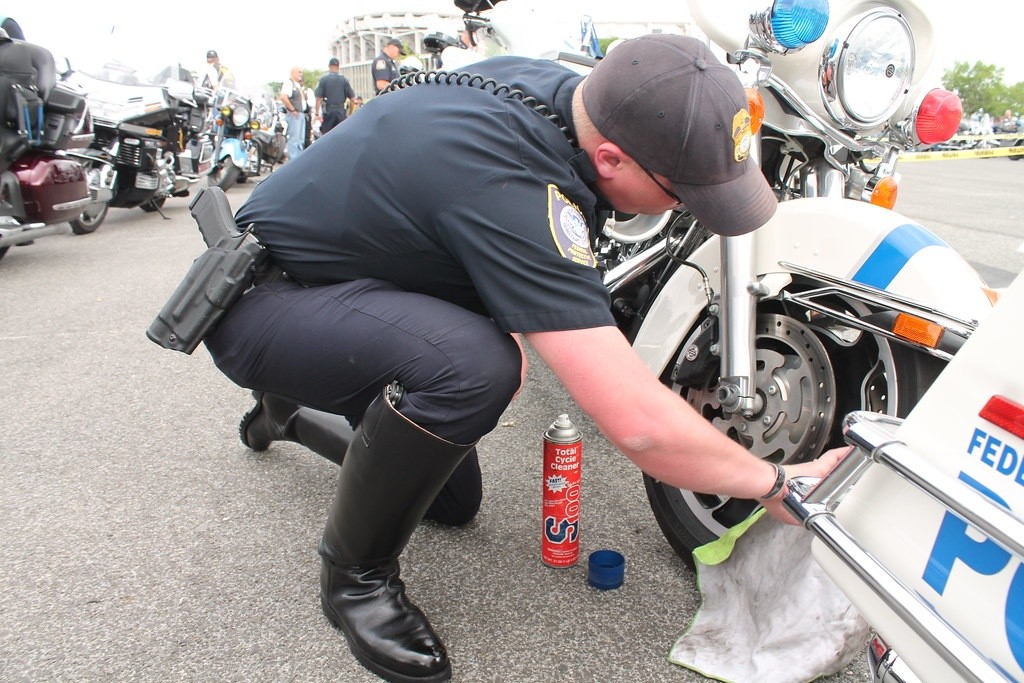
318, 387, 480, 683
239, 390, 360, 466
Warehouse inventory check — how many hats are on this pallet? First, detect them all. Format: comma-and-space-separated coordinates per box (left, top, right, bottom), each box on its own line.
207, 50, 217, 58
329, 58, 339, 66
582, 34, 778, 238
388, 38, 406, 55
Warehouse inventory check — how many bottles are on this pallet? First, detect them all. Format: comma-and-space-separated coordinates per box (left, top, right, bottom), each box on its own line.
542, 414, 582, 568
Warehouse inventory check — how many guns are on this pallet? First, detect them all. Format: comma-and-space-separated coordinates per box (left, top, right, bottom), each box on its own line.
143, 184, 269, 357
322, 102, 327, 115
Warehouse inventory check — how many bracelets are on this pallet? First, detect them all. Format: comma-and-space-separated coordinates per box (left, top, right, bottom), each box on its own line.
290, 110, 297, 112
760, 461, 786, 500
315, 114, 319, 116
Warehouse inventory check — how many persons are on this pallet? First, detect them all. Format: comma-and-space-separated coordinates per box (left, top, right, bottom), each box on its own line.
203, 48, 235, 144
202, 32, 853, 683
370, 39, 406, 94
280, 66, 317, 159
313, 57, 365, 138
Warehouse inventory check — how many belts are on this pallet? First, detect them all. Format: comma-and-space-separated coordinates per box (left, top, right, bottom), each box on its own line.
326, 104, 344, 108
